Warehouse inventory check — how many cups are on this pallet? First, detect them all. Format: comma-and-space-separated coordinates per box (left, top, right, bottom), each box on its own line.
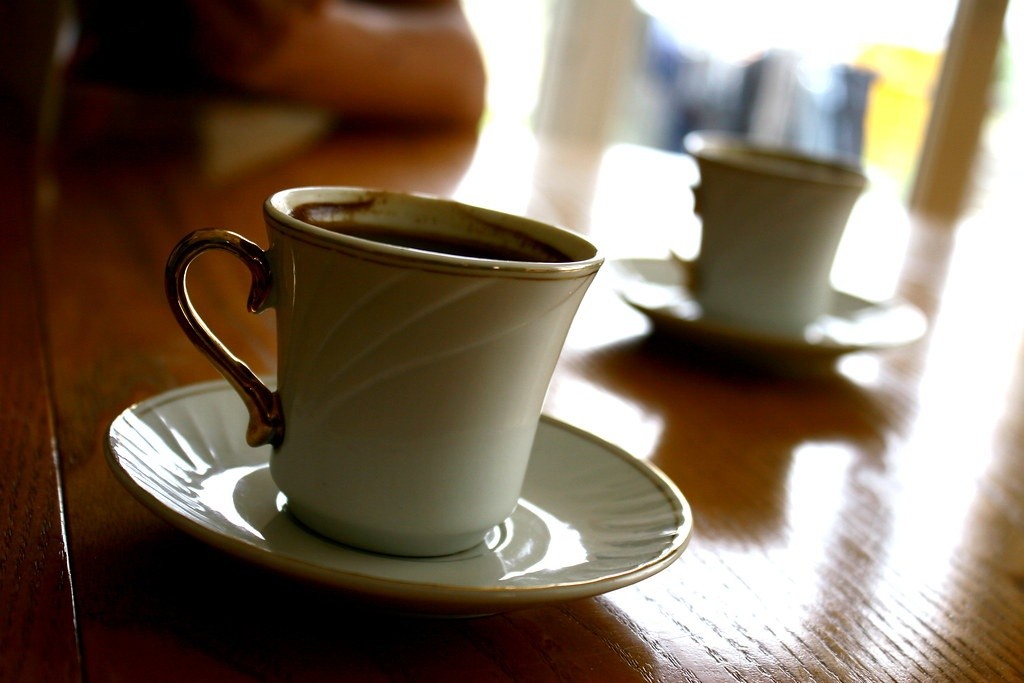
662, 130, 874, 334
163, 184, 605, 559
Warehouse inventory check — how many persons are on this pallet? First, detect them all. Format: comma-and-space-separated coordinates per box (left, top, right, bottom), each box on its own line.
0, 2, 485, 129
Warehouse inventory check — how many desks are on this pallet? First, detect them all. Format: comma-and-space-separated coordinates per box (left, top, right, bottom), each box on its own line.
0, 68, 1024, 683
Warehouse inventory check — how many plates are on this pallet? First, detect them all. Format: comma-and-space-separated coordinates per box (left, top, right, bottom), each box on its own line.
101, 365, 694, 622
605, 249, 929, 370
